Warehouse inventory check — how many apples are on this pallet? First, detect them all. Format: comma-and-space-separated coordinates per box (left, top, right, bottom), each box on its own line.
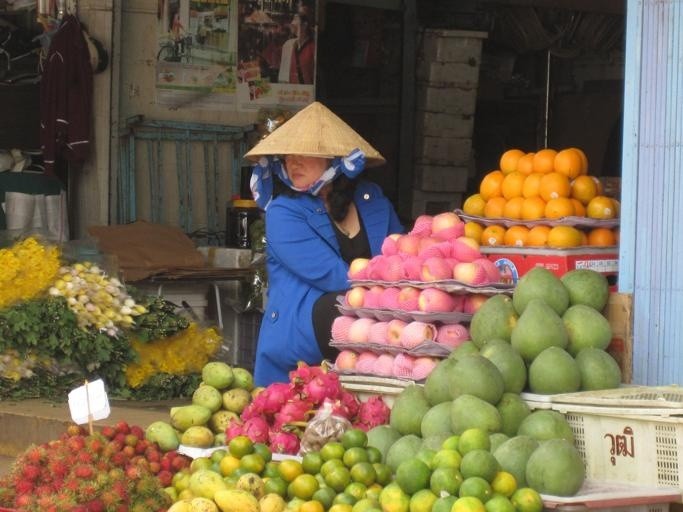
331, 211, 502, 382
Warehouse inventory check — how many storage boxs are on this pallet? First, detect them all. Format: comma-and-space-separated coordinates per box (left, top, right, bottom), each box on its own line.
411, 27, 489, 220
483, 246, 618, 295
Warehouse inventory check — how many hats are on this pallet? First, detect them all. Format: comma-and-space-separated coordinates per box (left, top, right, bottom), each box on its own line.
243, 101, 387, 169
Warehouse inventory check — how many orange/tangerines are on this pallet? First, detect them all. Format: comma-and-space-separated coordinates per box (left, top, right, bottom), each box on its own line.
163, 430, 545, 512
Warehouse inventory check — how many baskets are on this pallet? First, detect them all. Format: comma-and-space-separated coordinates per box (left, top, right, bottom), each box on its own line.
548, 383, 683, 512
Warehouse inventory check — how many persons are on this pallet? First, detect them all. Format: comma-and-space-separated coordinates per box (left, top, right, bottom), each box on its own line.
242, 101, 406, 387
237, 4, 314, 86
170, 12, 186, 55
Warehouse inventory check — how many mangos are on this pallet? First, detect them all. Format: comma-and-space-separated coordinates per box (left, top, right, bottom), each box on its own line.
146, 361, 268, 452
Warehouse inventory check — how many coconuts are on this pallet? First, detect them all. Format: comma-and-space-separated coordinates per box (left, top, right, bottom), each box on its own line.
367, 266, 621, 498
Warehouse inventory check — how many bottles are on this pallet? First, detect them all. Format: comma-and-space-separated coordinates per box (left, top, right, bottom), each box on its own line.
226, 197, 262, 254
